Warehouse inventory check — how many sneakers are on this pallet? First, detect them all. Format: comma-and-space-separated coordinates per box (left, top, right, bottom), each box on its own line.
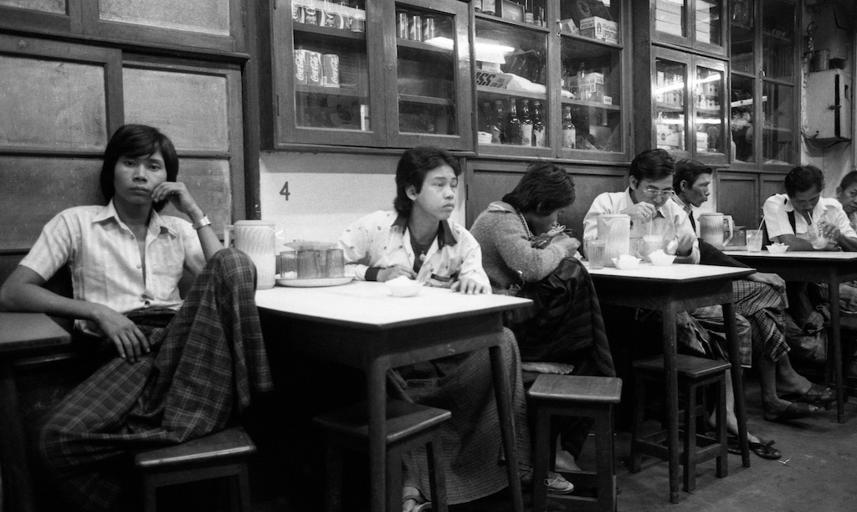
507, 463, 575, 495
554, 449, 583, 471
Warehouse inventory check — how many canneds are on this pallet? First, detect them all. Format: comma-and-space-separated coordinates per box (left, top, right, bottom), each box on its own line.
291, 0, 365, 34
397, 13, 435, 42
295, 48, 339, 88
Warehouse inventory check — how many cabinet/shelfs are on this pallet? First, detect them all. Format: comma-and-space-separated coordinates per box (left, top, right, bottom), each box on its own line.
265, 2, 478, 160
476, 2, 632, 171
731, 3, 799, 172
633, 2, 731, 170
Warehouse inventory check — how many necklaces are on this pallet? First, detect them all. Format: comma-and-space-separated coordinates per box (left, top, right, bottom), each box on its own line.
418, 249, 428, 262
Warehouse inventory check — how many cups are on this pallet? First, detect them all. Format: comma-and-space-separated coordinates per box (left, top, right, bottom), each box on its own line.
644, 236, 663, 263
275, 250, 344, 280
745, 229, 762, 251
813, 48, 830, 72
586, 240, 605, 270
806, 223, 827, 249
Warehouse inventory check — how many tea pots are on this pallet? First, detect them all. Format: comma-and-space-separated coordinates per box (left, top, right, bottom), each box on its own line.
223, 218, 281, 289
598, 214, 630, 267
697, 212, 734, 250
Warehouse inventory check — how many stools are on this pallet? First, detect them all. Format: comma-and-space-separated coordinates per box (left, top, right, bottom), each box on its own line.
520, 358, 576, 384
312, 393, 454, 509
627, 346, 733, 494
527, 372, 624, 508
136, 423, 258, 508
821, 317, 854, 411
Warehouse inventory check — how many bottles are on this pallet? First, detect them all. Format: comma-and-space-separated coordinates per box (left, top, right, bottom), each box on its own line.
482, 97, 576, 150
524, 9, 534, 27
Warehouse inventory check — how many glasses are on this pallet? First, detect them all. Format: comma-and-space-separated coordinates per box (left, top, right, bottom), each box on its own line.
638, 181, 675, 199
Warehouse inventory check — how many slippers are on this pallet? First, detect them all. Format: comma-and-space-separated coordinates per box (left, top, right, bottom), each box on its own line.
762, 401, 820, 423
751, 441, 783, 461
780, 382, 838, 405
704, 422, 751, 456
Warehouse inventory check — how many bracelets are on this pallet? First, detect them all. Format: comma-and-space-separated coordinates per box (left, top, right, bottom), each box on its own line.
192, 212, 212, 230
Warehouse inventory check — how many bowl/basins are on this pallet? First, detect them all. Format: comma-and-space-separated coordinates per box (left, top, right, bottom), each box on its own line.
610, 257, 641, 268
765, 244, 790, 253
649, 255, 677, 266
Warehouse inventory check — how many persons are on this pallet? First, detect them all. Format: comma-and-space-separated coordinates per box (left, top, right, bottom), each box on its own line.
328, 145, 577, 512
671, 157, 837, 422
466, 162, 623, 474
835, 169, 857, 288
582, 149, 760, 449
762, 165, 857, 382
0, 123, 275, 511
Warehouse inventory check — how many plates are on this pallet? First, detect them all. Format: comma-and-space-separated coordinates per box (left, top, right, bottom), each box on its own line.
276, 275, 355, 289
